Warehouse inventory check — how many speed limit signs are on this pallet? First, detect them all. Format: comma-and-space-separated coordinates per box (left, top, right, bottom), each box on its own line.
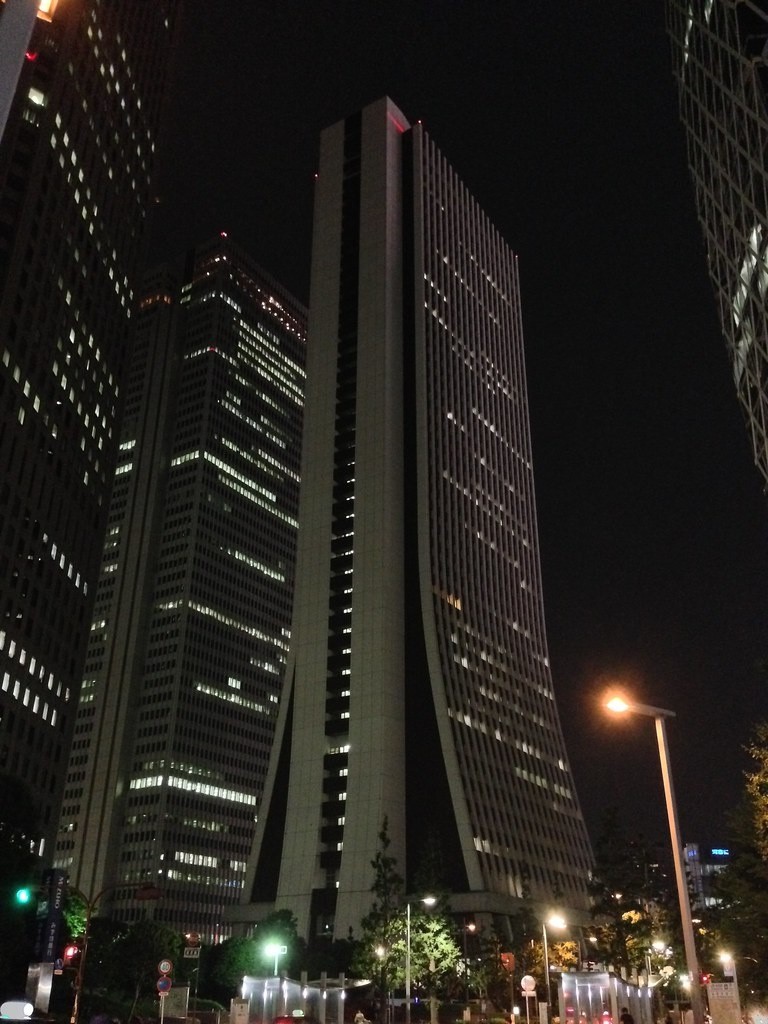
158, 959, 173, 976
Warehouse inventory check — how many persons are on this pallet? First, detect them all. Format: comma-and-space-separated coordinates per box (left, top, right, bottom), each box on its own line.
354, 1010, 371, 1024
619, 1008, 634, 1024
685, 1004, 694, 1024
665, 1004, 680, 1024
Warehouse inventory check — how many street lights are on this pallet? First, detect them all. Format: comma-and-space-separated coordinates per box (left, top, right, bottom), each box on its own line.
16, 882, 166, 1024
593, 683, 707, 1024
239, 893, 745, 1024
